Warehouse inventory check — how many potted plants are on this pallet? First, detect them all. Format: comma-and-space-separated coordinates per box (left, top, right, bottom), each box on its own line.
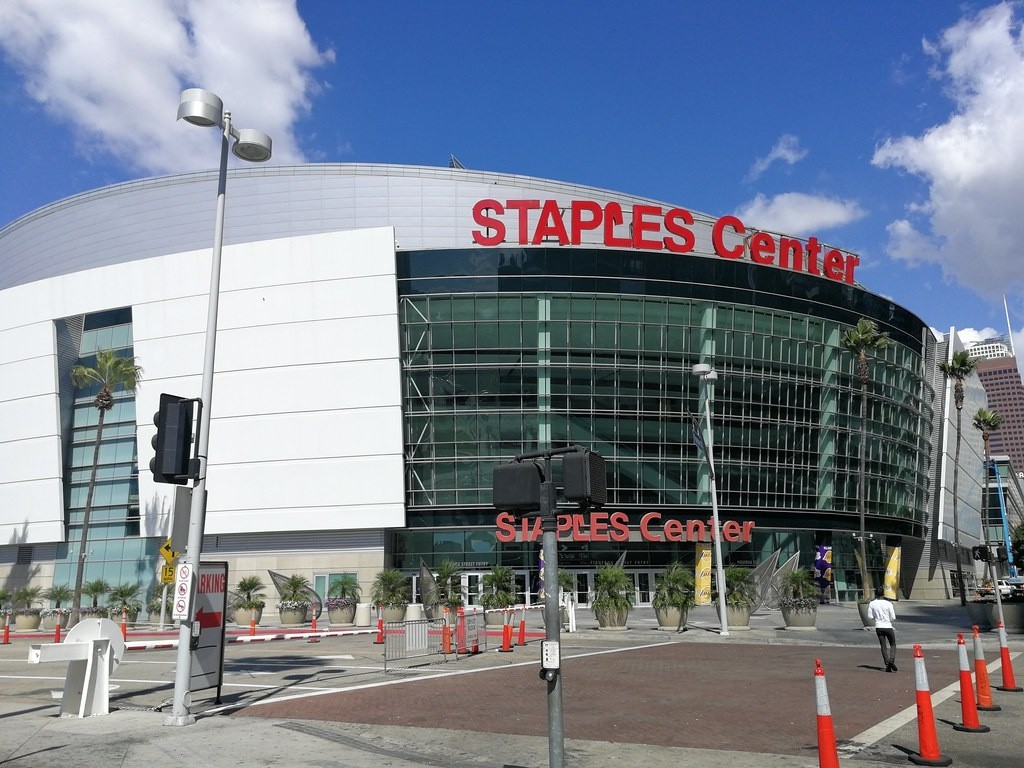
857, 599, 874, 632
236, 575, 266, 628
537, 570, 573, 629
715, 565, 752, 630
478, 565, 517, 629
592, 564, 636, 631
652, 561, 697, 631
966, 598, 994, 630
430, 560, 462, 628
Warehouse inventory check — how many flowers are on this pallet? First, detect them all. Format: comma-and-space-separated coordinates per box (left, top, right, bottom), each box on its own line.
275, 574, 312, 610
325, 574, 364, 609
42, 583, 71, 615
147, 586, 172, 615
778, 569, 820, 615
12, 587, 41, 614
110, 583, 142, 614
0, 589, 12, 616
79, 579, 109, 613
370, 570, 409, 608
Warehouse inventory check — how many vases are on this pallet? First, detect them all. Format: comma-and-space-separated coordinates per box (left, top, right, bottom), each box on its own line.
780, 604, 818, 631
42, 614, 68, 632
376, 606, 406, 627
329, 608, 356, 627
280, 611, 308, 628
0, 617, 6, 632
14, 615, 41, 633
150, 615, 174, 630
985, 601, 1024, 633
79, 608, 108, 621
112, 614, 137, 631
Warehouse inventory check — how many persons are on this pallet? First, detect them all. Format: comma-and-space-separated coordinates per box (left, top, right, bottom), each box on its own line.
868, 587, 898, 672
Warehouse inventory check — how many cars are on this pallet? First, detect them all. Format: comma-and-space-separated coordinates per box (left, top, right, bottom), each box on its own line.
976, 574, 1024, 598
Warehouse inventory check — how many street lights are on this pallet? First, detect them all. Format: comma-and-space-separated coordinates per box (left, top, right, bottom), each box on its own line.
165, 91, 274, 724
692, 363, 730, 636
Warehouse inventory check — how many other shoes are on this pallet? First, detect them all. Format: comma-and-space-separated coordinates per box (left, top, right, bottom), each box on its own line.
888, 660, 898, 671
886, 667, 892, 673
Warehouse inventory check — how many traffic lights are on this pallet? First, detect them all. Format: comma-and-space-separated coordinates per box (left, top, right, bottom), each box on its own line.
972, 546, 988, 560
562, 450, 608, 506
